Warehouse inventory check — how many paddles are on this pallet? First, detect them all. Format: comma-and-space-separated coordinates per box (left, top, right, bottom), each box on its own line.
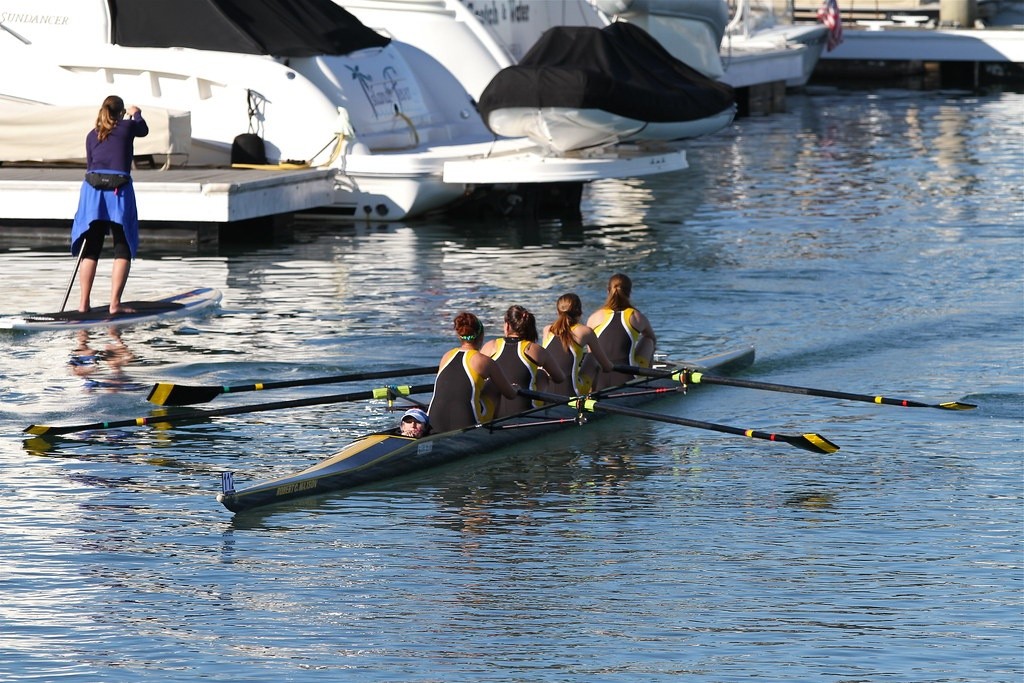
20, 383, 434, 438
584, 361, 979, 412
57, 116, 131, 314
517, 388, 841, 456
144, 364, 441, 407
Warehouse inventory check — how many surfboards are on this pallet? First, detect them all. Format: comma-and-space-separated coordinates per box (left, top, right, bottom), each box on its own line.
0, 286, 222, 332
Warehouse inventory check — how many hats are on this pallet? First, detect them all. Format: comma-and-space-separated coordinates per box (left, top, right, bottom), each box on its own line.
401, 408, 430, 425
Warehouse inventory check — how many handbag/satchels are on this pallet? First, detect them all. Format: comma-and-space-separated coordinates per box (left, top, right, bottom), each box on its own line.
85, 173, 131, 190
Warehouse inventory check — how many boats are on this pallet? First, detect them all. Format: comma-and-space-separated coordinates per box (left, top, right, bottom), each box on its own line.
0, 0, 835, 220
217, 344, 755, 514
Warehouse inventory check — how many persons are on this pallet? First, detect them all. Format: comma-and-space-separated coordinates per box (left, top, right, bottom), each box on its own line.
426, 312, 521, 439
479, 305, 566, 424
71, 96, 149, 314
399, 408, 432, 441
541, 294, 615, 408
588, 274, 657, 394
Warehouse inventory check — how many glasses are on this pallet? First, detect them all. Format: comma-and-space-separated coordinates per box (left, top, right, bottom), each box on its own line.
118, 108, 126, 118
